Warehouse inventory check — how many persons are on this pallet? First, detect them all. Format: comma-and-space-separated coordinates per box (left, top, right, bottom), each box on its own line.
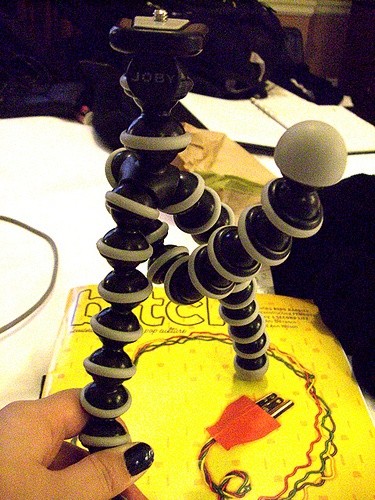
0, 387, 156, 499
